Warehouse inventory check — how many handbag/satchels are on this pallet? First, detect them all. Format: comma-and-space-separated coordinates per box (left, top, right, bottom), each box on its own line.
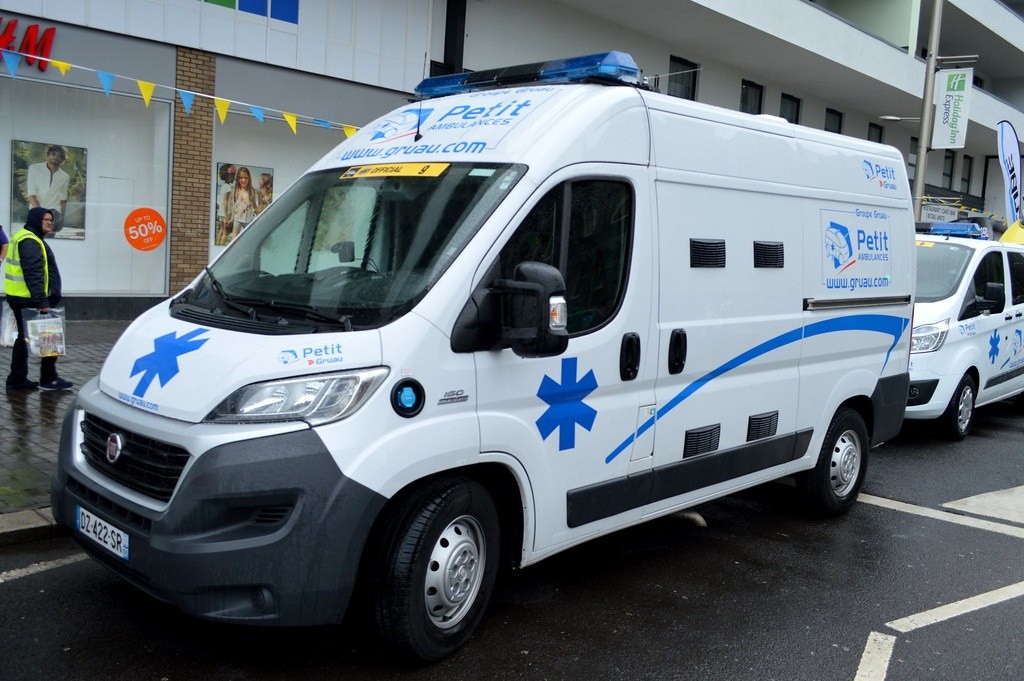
0, 300, 18, 347
22, 306, 67, 357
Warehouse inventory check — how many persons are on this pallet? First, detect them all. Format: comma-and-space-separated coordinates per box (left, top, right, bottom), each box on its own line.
27, 146, 70, 238
216, 164, 273, 247
3, 208, 73, 391
0, 226, 9, 319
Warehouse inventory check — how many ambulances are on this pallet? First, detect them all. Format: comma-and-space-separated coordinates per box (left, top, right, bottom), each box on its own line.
49, 43, 915, 662
902, 222, 1024, 440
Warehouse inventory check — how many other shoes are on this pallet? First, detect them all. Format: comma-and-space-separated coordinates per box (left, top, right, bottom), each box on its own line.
6, 376, 39, 389
41, 378, 73, 390
